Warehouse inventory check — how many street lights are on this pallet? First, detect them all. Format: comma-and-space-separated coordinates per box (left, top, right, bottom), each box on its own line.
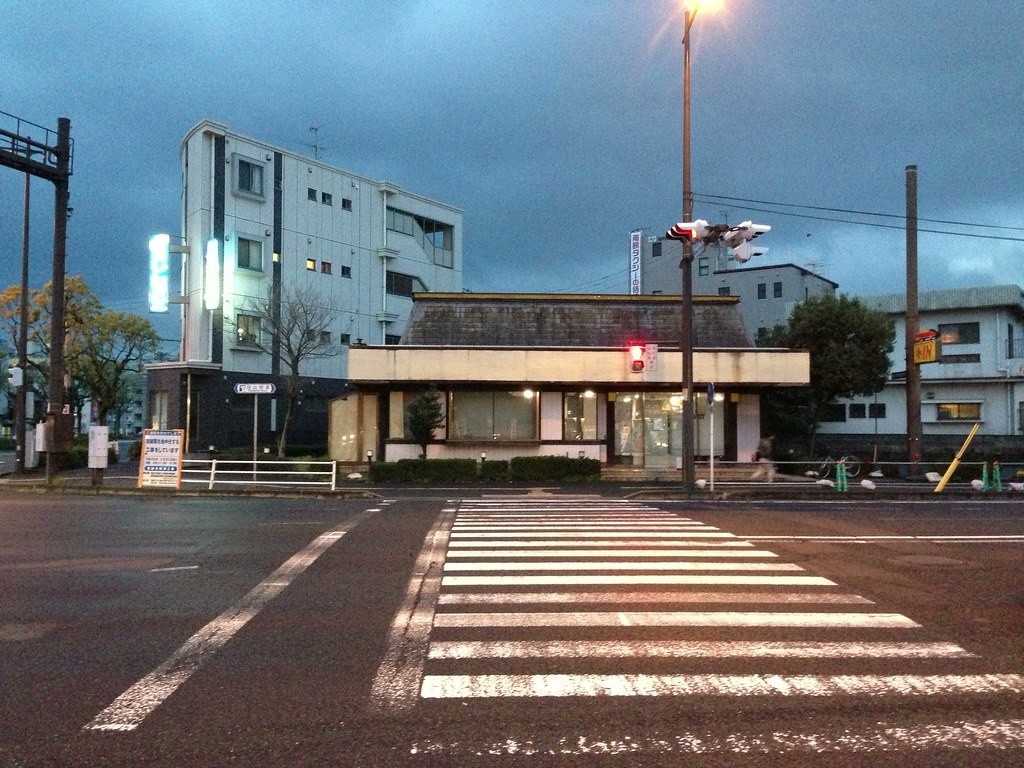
677, 0, 725, 498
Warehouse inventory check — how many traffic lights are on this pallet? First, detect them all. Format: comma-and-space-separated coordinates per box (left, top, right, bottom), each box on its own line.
734, 247, 771, 263
665, 217, 708, 246
630, 346, 644, 373
724, 220, 771, 243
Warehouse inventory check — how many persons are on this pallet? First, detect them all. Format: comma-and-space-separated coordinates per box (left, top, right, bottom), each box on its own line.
749, 432, 775, 483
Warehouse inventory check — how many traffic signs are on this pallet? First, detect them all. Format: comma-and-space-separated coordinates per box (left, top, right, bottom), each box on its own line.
233, 382, 277, 395
